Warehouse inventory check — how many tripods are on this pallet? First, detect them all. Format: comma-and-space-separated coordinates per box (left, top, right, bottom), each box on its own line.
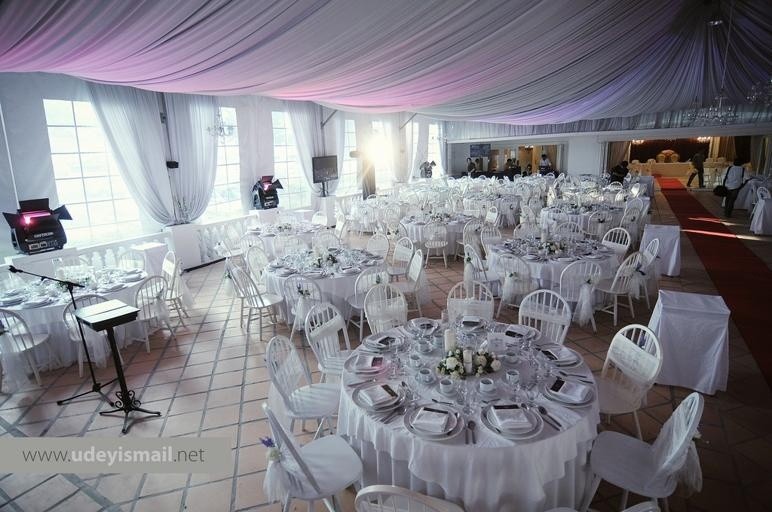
23, 271, 118, 407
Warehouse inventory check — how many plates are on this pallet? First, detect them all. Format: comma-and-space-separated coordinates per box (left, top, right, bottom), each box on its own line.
493, 235, 612, 265
547, 201, 618, 215
342, 315, 599, 444
0, 266, 148, 310
267, 245, 382, 279
402, 211, 471, 226
246, 221, 325, 235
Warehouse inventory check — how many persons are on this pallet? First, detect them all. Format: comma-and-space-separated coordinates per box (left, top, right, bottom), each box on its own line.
721, 156, 747, 218
464, 153, 553, 177
685, 147, 707, 189
609, 160, 629, 186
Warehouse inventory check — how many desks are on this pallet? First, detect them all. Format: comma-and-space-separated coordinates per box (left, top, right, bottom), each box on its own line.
641, 288, 732, 397
632, 162, 692, 177
136, 242, 169, 278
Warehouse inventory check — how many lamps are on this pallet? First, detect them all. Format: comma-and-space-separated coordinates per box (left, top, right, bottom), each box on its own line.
2, 198, 73, 256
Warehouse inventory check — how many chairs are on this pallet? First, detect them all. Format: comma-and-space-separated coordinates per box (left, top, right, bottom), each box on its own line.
0, 249, 190, 395
700, 158, 772, 221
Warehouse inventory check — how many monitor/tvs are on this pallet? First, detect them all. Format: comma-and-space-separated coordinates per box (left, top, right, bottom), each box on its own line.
312, 156, 338, 183
